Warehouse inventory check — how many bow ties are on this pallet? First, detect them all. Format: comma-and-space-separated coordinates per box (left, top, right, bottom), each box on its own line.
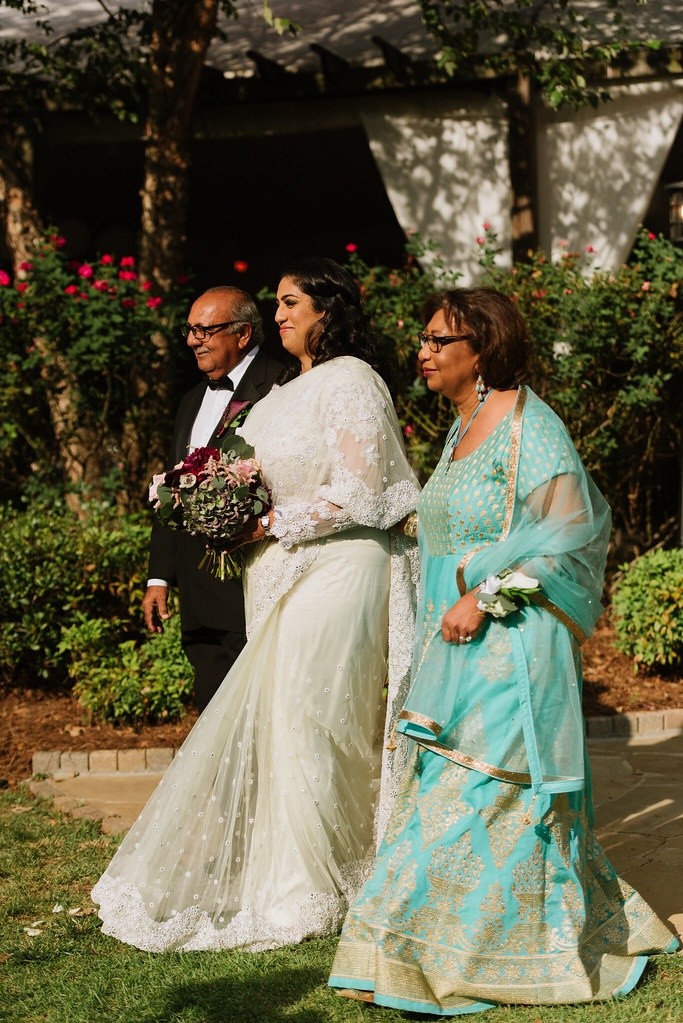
208, 376, 234, 391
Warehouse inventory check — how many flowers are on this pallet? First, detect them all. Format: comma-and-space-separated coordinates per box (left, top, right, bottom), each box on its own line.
153, 439, 269, 581
474, 570, 542, 617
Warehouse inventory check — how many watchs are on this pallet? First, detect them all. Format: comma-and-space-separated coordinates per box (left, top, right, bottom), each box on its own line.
260, 514, 273, 537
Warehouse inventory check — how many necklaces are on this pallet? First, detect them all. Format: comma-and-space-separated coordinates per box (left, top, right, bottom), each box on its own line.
460, 635, 472, 642
453, 387, 494, 447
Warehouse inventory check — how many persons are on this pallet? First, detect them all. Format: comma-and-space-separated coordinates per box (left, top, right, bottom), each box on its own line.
91, 260, 419, 953
335, 288, 677, 1016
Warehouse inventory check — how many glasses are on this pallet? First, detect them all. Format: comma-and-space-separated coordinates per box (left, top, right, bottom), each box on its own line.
418, 330, 472, 352
179, 320, 235, 339
